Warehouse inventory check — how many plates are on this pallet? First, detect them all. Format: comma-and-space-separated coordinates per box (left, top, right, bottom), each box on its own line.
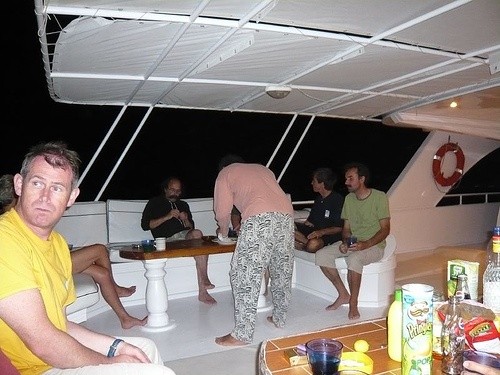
218, 241, 236, 245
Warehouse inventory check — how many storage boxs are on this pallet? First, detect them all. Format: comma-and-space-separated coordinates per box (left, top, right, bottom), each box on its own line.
284, 348, 308, 366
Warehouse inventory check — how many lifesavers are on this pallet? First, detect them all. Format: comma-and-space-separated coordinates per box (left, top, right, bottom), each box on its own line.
432, 142, 465, 187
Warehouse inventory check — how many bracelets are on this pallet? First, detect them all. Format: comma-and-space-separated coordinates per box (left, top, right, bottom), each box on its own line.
107, 338, 124, 357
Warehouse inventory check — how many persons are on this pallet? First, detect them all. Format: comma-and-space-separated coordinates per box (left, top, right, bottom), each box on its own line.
315, 161, 391, 319
0, 175, 148, 328
231, 206, 268, 296
293, 167, 344, 253
214, 157, 297, 347
0, 142, 175, 375
141, 179, 217, 306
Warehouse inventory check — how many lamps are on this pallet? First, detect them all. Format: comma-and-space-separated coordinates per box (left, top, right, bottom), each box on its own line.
265, 86, 292, 99
382, 107, 500, 140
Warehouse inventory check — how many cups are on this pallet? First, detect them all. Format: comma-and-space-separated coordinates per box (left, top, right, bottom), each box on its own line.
462, 350, 500, 373
153, 238, 166, 251
142, 240, 154, 252
305, 339, 343, 375
347, 237, 357, 248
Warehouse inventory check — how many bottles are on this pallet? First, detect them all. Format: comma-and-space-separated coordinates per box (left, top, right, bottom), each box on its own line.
387, 289, 402, 362
455, 274, 470, 300
401, 283, 434, 375
486, 206, 500, 263
482, 226, 500, 317
441, 296, 464, 375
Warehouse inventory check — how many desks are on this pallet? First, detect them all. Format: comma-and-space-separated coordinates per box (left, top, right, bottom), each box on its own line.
120, 236, 274, 333
257, 297, 484, 375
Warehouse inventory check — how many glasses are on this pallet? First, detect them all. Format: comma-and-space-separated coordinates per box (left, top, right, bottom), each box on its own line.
168, 189, 181, 194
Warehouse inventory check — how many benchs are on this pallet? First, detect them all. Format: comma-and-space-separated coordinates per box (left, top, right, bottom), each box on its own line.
53, 197, 271, 325
291, 233, 398, 308
